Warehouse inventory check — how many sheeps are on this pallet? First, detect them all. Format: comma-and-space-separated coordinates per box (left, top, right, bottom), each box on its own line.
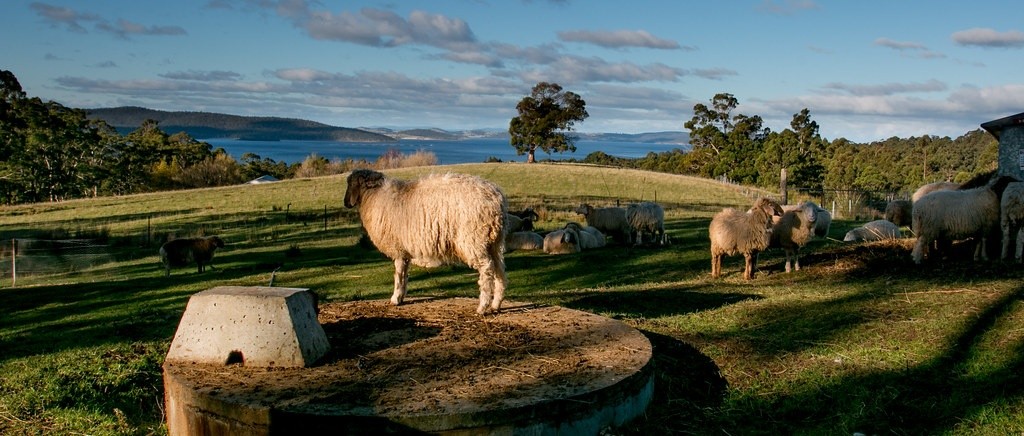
158, 236, 225, 277
709, 197, 831, 283
843, 176, 1024, 263
343, 169, 507, 315
508, 200, 673, 255
